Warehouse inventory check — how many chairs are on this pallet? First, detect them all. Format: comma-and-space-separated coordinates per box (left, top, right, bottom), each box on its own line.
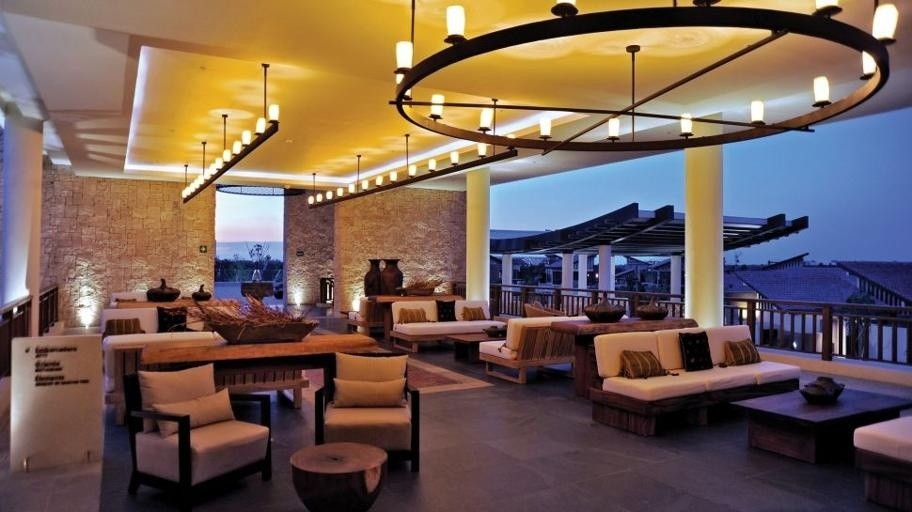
122, 365, 271, 508
315, 351, 419, 478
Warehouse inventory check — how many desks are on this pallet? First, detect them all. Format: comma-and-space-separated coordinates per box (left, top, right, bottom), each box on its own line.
289, 442, 388, 512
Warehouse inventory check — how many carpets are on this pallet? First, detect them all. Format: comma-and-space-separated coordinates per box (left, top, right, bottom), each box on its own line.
301, 327, 496, 403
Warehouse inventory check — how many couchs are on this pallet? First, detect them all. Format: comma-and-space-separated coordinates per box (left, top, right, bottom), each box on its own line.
388, 297, 629, 385
101, 292, 309, 425
587, 325, 801, 435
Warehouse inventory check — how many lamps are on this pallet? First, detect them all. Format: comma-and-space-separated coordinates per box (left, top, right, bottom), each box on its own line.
385, 0, 901, 158
301, 133, 517, 211
180, 63, 281, 204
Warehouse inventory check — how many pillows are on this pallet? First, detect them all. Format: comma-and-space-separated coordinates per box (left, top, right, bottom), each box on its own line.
618, 347, 664, 378
724, 336, 762, 365
334, 352, 409, 409
679, 331, 715, 372
136, 363, 237, 437
